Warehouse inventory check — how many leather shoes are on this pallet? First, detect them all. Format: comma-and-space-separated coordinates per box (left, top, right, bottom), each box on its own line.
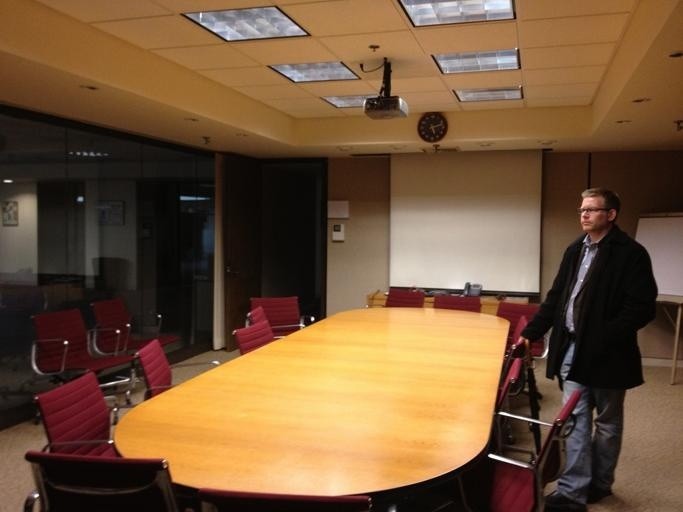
542, 490, 588, 512
586, 484, 614, 504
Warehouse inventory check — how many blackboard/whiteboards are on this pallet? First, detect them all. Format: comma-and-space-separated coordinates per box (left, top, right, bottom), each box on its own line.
634, 213, 683, 304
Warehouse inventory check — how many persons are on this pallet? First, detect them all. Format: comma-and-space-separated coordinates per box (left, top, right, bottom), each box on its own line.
518, 187, 658, 511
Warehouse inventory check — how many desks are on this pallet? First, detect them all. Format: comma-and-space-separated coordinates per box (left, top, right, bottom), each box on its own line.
112, 305, 511, 511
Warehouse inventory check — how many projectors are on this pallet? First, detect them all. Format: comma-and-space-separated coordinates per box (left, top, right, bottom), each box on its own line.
363, 97, 407, 120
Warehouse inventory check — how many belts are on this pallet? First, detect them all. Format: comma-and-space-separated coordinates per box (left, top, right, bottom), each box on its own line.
567, 334, 577, 342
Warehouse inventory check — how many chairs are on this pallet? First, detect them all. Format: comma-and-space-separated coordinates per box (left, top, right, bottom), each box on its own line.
34, 370, 119, 457
496, 302, 547, 359
433, 294, 480, 314
23, 450, 179, 512
246, 306, 306, 339
136, 338, 221, 402
385, 289, 425, 309
467, 388, 581, 512
495, 357, 523, 414
89, 297, 182, 406
30, 307, 132, 426
232, 320, 277, 356
244, 296, 305, 337
197, 488, 372, 511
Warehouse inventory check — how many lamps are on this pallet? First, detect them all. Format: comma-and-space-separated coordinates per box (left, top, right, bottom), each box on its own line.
418, 113, 448, 143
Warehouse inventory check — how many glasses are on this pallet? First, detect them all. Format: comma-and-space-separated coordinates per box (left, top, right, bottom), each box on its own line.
575, 206, 608, 214
